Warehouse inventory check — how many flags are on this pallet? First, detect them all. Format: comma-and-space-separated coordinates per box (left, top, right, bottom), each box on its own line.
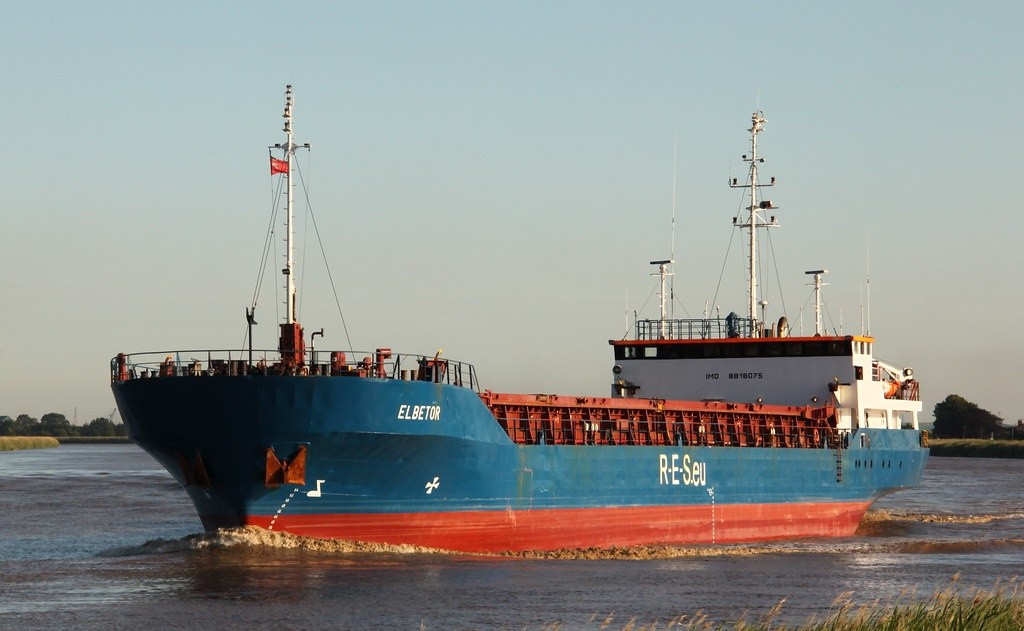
271, 157, 289, 175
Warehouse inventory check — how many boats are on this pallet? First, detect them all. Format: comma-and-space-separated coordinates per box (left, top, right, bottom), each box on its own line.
107, 83, 932, 559
